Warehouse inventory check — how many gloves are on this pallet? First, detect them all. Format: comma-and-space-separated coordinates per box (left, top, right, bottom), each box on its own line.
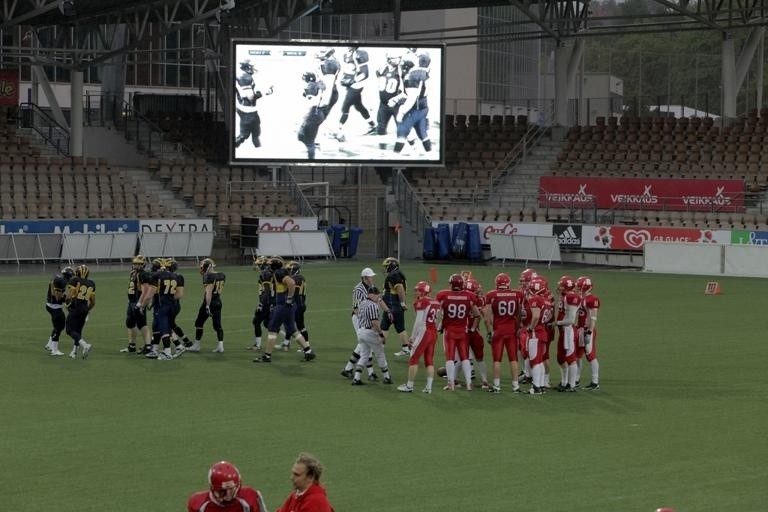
584, 331, 592, 344
206, 305, 213, 317
256, 303, 274, 313
134, 303, 152, 314
286, 295, 294, 307
466, 328, 474, 333
487, 333, 492, 344
547, 321, 557, 328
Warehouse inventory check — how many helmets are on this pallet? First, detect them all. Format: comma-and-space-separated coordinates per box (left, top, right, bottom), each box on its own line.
132, 254, 177, 271
61, 265, 89, 279
415, 280, 432, 294
495, 269, 593, 294
208, 461, 241, 506
255, 255, 300, 277
449, 275, 480, 292
361, 258, 399, 294
200, 258, 216, 274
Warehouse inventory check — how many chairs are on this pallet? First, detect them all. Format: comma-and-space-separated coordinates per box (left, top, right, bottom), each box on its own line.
403, 109, 768, 233
0, 112, 305, 246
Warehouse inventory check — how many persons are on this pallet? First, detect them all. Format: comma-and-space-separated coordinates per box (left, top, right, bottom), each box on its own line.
235, 44, 431, 160
274, 452, 335, 512
342, 258, 601, 394
44, 267, 76, 356
120, 255, 194, 360
245, 256, 315, 363
65, 264, 96, 359
185, 259, 226, 353
188, 461, 268, 512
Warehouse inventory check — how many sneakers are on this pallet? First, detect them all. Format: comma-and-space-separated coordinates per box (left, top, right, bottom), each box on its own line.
44, 343, 92, 359
341, 366, 391, 386
213, 346, 224, 353
422, 387, 432, 394
394, 350, 410, 356
397, 384, 414, 392
120, 340, 200, 360
246, 342, 315, 362
442, 369, 500, 394
513, 370, 600, 394
